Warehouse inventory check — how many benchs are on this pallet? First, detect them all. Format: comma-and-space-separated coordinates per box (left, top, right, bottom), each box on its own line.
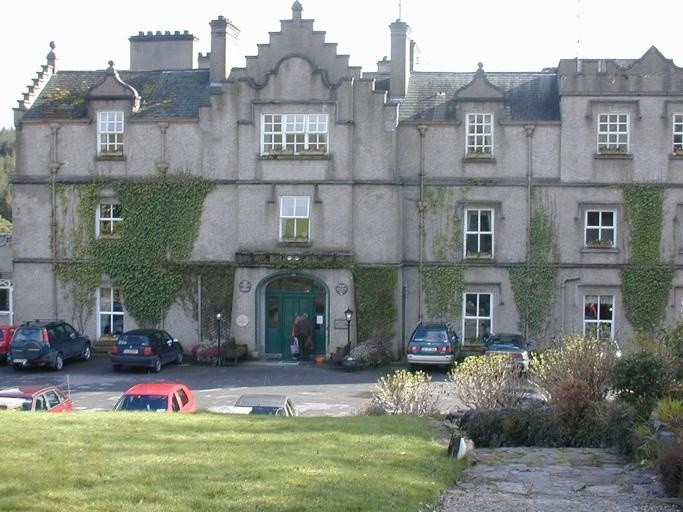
221, 336, 247, 366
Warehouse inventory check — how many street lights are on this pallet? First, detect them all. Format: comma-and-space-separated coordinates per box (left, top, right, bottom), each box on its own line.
344, 306, 353, 356
216, 310, 221, 368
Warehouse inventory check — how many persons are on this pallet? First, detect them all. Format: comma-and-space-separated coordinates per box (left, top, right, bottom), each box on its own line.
586, 302, 596, 317
293, 312, 314, 361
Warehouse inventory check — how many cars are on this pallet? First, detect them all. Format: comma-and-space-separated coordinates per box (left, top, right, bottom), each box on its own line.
0, 382, 295, 416
406, 322, 538, 377
0, 319, 185, 373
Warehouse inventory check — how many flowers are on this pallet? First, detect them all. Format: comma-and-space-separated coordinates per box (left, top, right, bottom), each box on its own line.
191, 342, 221, 359
